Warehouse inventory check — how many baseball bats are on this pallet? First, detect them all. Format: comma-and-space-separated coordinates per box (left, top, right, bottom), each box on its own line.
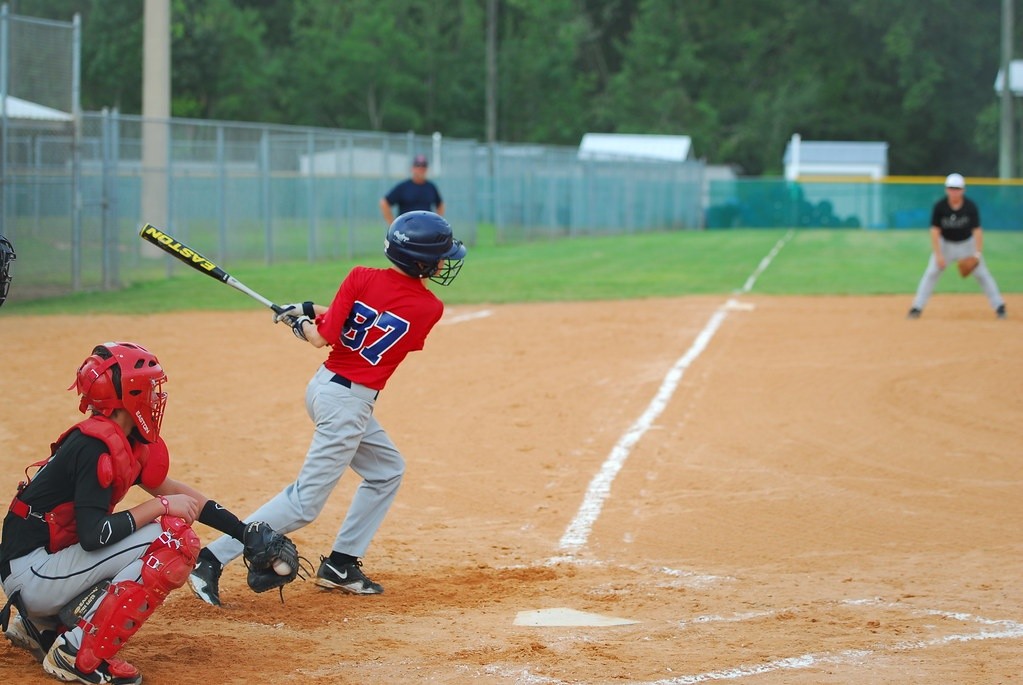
138, 222, 298, 325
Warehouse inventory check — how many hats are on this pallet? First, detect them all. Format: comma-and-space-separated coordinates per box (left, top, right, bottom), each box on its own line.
945, 173, 966, 189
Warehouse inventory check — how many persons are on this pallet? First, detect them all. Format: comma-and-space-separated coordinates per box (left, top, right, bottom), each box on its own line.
187, 211, 467, 608
0, 341, 317, 685
910, 173, 1012, 317
381, 154, 445, 227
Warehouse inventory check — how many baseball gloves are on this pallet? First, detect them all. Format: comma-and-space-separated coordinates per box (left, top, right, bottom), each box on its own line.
957, 256, 980, 277
243, 521, 299, 593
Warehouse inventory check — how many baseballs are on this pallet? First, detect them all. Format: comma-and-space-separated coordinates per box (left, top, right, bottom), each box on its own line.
272, 557, 292, 575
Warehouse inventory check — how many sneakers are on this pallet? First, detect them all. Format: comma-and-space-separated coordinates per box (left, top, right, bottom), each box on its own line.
42, 631, 143, 685
3, 611, 70, 663
188, 547, 221, 611
314, 554, 384, 596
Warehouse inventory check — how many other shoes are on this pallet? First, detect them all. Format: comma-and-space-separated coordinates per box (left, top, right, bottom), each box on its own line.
998, 312, 1006, 319
908, 309, 920, 320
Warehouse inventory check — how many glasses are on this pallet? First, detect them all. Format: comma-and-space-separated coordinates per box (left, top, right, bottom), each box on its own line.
414, 162, 427, 167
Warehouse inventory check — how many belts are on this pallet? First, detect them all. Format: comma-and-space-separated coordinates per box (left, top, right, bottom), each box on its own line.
0, 545, 55, 583
316, 364, 380, 402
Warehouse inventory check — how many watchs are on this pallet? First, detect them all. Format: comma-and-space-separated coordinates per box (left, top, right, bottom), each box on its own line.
157, 495, 169, 515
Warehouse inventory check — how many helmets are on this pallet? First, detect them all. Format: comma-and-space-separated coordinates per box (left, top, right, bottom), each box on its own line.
69, 342, 169, 446
384, 210, 467, 286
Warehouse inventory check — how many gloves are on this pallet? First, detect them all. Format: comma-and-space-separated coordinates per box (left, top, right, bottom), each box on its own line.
291, 314, 314, 342
272, 301, 316, 325
0, 591, 28, 632
